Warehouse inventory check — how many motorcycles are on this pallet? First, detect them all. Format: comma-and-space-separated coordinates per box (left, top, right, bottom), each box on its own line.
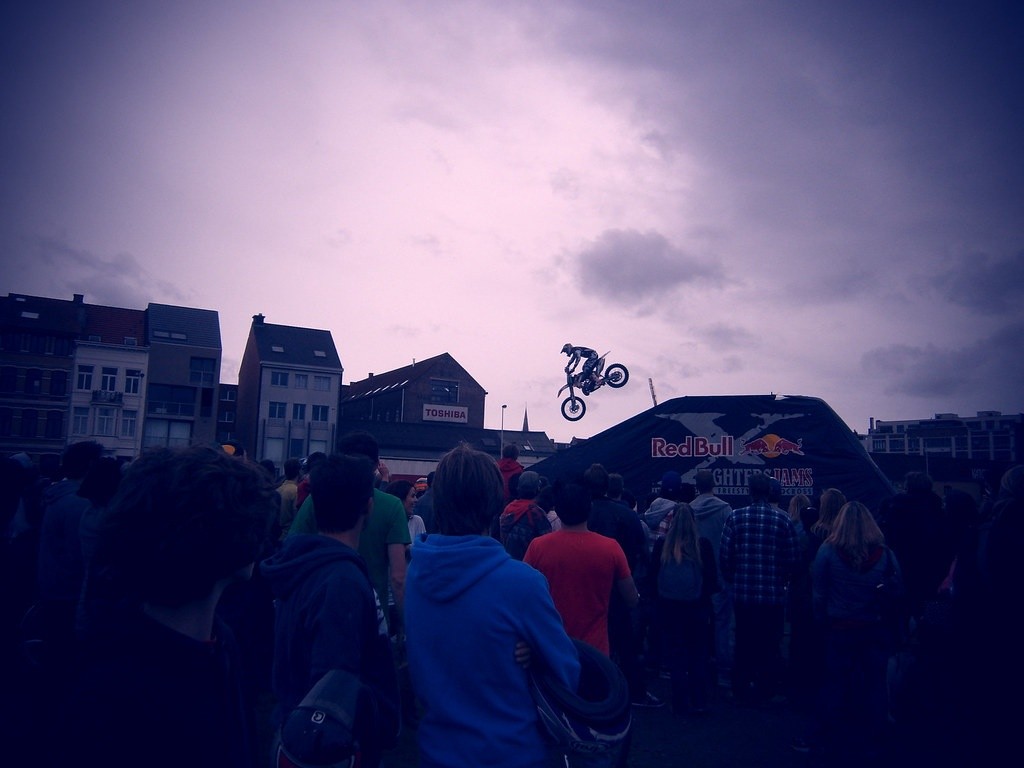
557, 350, 629, 421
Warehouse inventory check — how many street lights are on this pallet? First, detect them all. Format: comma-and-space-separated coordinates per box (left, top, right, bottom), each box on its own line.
501, 404, 507, 459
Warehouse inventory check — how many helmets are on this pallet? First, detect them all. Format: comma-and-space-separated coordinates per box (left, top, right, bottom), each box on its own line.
560, 342, 573, 357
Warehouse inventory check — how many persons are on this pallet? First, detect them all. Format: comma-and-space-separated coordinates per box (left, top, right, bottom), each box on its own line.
0, 441, 1023, 767
560, 342, 605, 386
